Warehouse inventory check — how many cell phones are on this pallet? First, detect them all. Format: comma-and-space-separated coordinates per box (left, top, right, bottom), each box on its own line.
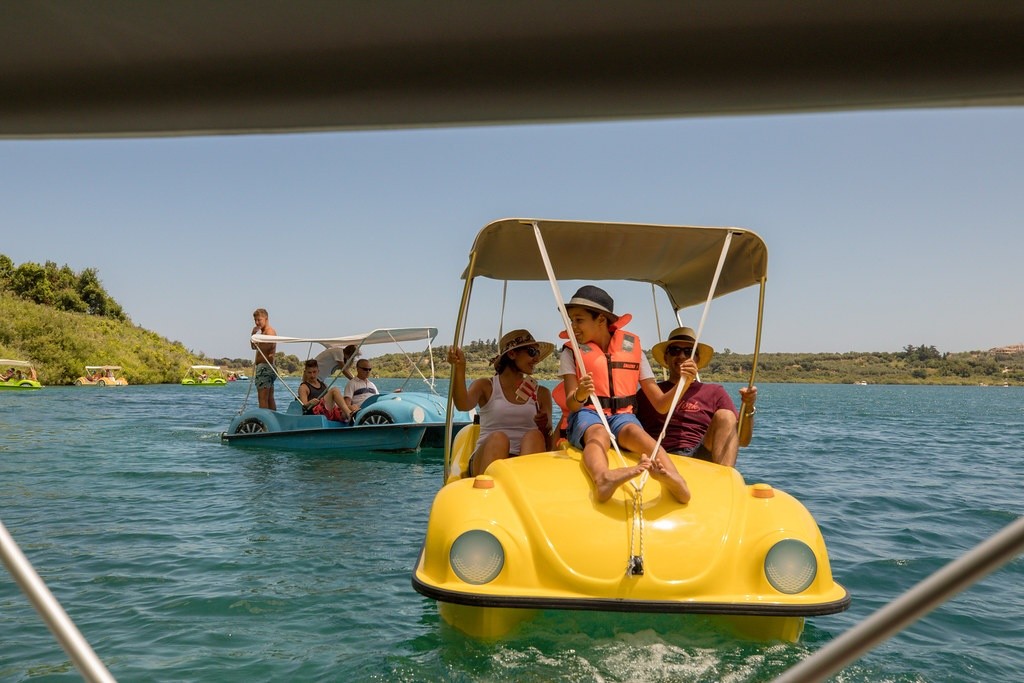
516, 376, 538, 401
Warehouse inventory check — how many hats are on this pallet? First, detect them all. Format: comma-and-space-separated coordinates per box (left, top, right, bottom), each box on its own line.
488, 328, 555, 373
557, 285, 619, 324
652, 327, 714, 369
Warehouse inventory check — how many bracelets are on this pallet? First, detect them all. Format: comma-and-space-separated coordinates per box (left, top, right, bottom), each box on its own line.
573, 388, 587, 404
744, 406, 755, 417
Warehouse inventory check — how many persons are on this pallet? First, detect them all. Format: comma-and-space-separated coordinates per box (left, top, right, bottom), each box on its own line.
198, 371, 207, 380
447, 329, 552, 477
301, 344, 362, 383
92, 369, 115, 380
298, 359, 380, 422
250, 308, 276, 410
636, 327, 758, 468
558, 285, 698, 504
0, 368, 32, 382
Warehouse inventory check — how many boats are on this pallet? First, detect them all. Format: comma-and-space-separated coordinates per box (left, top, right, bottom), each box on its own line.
226, 370, 248, 381
862, 380, 866, 385
73, 365, 128, 387
221, 327, 479, 453
411, 217, 851, 646
181, 364, 227, 385
0, 359, 42, 389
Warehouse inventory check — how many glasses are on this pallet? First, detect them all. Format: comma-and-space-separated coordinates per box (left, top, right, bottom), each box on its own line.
358, 367, 372, 371
520, 346, 541, 358
666, 345, 694, 357
